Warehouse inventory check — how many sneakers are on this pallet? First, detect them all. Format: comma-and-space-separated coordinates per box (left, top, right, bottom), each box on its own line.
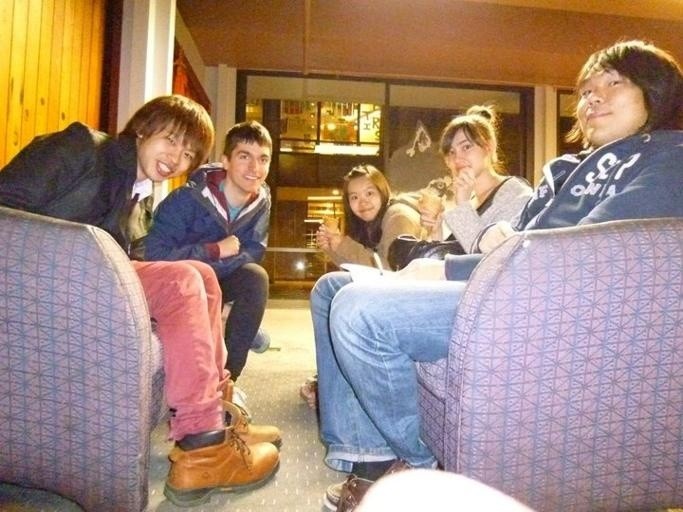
228, 302, 271, 354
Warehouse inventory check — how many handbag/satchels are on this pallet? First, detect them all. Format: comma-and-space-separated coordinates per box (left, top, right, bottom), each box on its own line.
387, 235, 466, 271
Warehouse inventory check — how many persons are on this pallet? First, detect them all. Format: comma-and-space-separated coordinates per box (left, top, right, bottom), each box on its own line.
309, 39, 683, 512
298, 165, 432, 409
0, 94, 283, 507
418, 102, 534, 262
128, 119, 272, 423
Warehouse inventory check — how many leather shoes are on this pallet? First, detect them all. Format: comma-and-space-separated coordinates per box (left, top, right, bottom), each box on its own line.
168, 379, 283, 448
164, 399, 281, 506
323, 460, 440, 512
336, 457, 416, 512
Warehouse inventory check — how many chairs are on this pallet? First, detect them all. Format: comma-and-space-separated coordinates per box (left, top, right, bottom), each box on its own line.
417, 216, 683, 512
0, 204, 166, 512
